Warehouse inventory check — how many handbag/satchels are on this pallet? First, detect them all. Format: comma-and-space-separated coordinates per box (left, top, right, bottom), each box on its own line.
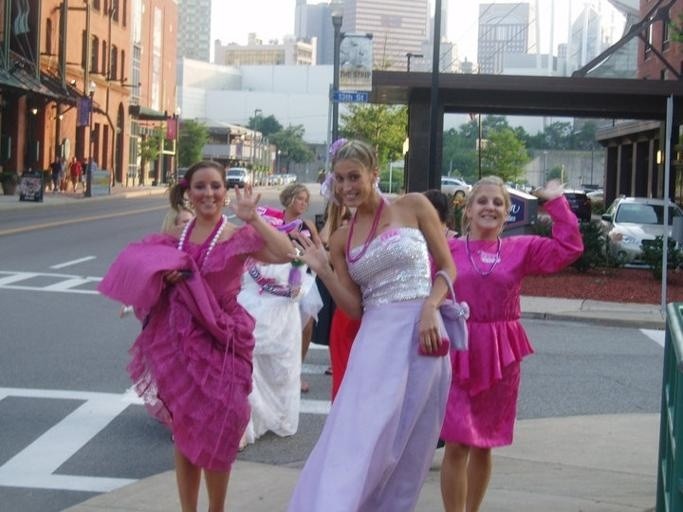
439, 300, 472, 352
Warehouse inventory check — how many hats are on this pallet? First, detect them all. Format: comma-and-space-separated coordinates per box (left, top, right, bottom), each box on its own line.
258, 206, 302, 233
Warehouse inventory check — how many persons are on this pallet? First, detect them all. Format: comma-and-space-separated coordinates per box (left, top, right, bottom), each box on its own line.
435, 176, 584, 512
163, 206, 193, 230
50, 153, 93, 194
237, 183, 362, 450
283, 138, 459, 512
96, 161, 297, 512
425, 190, 465, 240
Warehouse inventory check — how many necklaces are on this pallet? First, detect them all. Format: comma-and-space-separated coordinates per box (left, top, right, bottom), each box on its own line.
465, 235, 502, 277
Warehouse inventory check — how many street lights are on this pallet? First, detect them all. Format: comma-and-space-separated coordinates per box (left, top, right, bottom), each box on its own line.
327, 0, 345, 142
172, 105, 182, 182
85, 78, 98, 197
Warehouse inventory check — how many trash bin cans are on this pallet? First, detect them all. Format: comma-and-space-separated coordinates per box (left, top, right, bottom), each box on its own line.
565, 193, 592, 225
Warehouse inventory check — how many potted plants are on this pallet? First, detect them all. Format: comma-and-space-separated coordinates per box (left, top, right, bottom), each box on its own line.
0, 171, 21, 195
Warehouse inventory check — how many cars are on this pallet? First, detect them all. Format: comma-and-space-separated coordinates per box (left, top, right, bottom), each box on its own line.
561, 186, 594, 223
226, 167, 297, 189
440, 175, 474, 200
598, 191, 683, 271
